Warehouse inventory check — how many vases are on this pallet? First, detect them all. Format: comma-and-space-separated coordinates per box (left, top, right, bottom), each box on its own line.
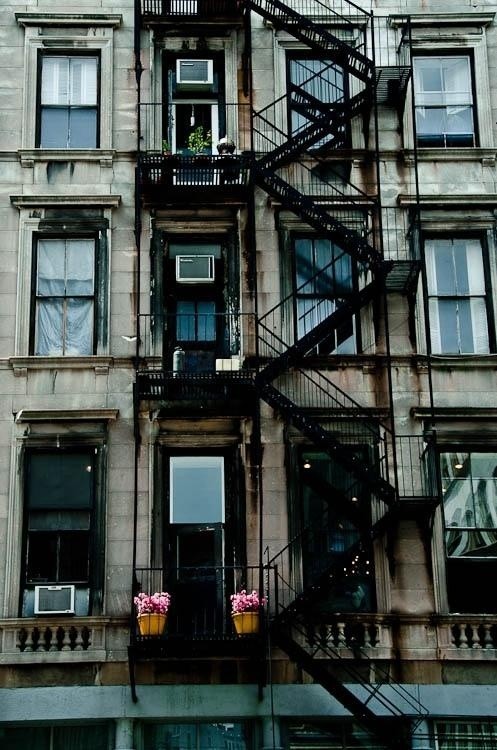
231, 610, 259, 634
137, 612, 167, 636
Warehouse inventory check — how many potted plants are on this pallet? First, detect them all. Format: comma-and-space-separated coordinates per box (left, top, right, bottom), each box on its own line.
188, 126, 213, 163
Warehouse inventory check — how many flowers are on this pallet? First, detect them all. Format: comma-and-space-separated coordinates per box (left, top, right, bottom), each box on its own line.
134, 591, 170, 615
229, 590, 268, 614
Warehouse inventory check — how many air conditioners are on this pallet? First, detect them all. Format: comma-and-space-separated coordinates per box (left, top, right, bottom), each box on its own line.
174, 253, 215, 284
33, 586, 77, 615
175, 57, 214, 85
